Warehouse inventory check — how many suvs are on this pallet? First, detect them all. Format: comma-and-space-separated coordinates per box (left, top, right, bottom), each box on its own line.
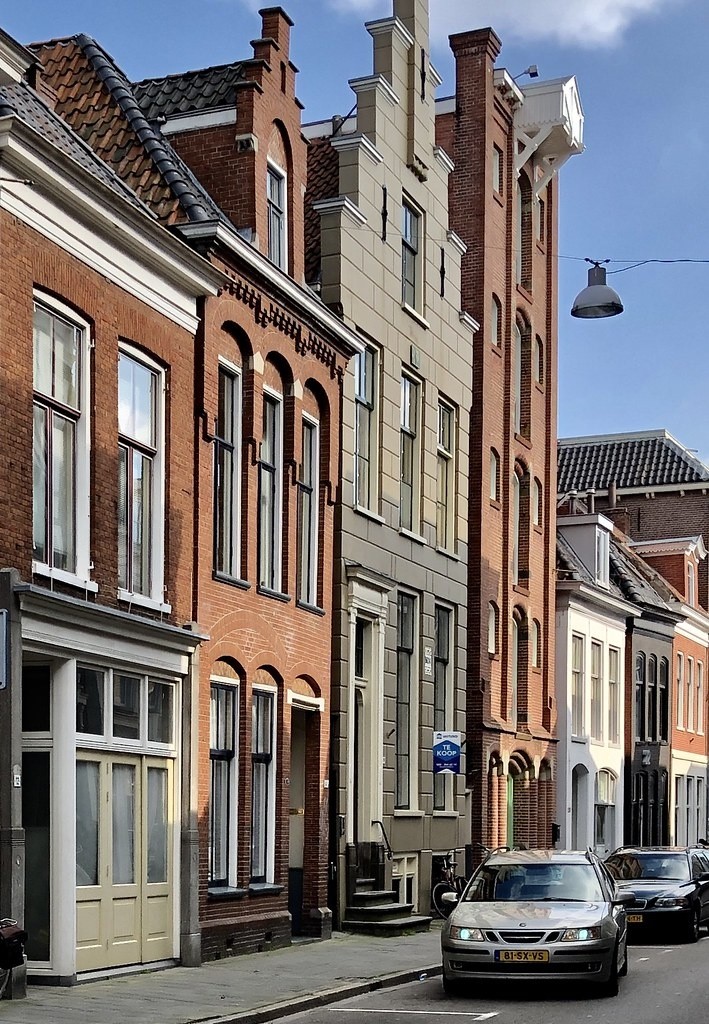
603, 844, 709, 943
441, 844, 637, 998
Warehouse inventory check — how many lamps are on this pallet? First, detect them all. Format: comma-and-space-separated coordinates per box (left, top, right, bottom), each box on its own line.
569, 258, 625, 320
513, 64, 539, 79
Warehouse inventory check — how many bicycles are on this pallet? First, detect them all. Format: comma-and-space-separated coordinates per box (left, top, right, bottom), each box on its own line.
433, 851, 471, 921
471, 843, 516, 873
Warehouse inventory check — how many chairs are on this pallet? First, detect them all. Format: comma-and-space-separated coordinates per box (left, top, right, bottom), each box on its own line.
510, 875, 526, 887
495, 882, 521, 899
543, 884, 569, 896
